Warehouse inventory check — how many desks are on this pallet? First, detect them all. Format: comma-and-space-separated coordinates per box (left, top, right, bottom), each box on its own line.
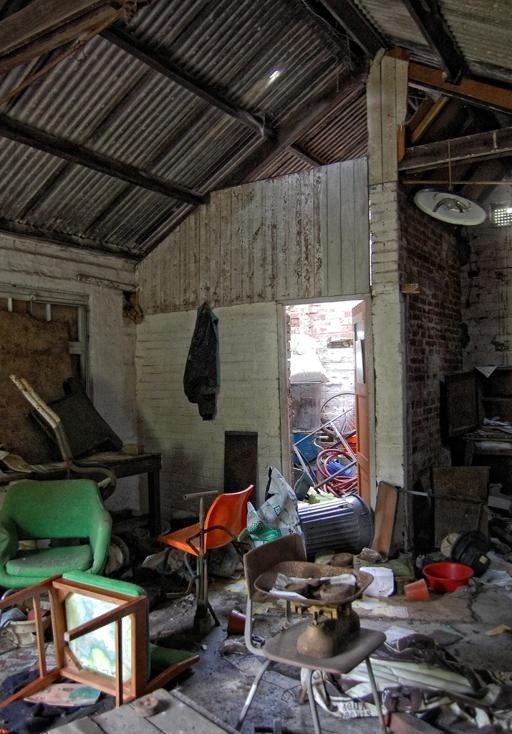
370, 481, 428, 564
0, 448, 162, 544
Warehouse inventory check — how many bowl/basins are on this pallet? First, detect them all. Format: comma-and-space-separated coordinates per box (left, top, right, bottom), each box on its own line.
422, 562, 474, 594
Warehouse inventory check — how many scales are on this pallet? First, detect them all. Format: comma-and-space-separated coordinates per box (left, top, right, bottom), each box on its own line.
253, 560, 374, 659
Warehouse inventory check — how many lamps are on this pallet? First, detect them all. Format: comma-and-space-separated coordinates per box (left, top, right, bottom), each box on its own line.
414, 141, 487, 225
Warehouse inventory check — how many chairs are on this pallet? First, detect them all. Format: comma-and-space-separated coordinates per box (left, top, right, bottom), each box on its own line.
1, 569, 200, 713
1, 476, 112, 588
230, 529, 386, 734
156, 482, 256, 625
